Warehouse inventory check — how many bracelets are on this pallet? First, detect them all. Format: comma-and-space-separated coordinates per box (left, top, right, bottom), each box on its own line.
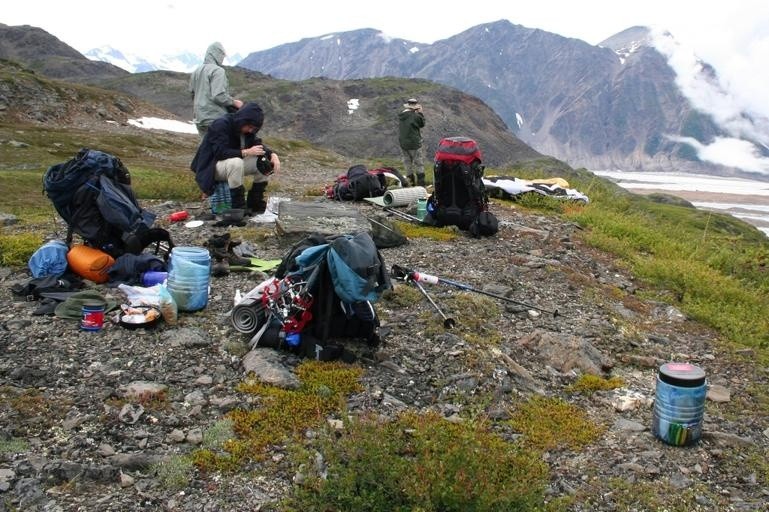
244, 149, 247, 158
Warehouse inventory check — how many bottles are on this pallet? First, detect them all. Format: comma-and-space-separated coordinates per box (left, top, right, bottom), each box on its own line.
139, 271, 168, 286
414, 272, 438, 284
171, 211, 188, 221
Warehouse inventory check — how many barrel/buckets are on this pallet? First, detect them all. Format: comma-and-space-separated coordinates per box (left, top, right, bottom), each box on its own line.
654, 363, 707, 445
168, 246, 212, 312
210, 181, 233, 215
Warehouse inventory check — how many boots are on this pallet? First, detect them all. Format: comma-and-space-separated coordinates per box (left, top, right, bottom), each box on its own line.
229, 185, 252, 217
416, 172, 425, 186
407, 173, 415, 186
248, 181, 267, 212
208, 233, 252, 277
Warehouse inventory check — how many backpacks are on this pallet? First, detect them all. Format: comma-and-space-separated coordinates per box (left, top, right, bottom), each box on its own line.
43, 148, 157, 256
426, 136, 498, 237
346, 163, 381, 197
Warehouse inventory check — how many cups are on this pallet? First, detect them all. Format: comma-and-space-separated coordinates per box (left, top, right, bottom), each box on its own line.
417, 198, 427, 218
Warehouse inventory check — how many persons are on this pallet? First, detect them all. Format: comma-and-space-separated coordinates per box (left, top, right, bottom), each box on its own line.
398, 98, 426, 187
188, 41, 244, 201
190, 100, 281, 214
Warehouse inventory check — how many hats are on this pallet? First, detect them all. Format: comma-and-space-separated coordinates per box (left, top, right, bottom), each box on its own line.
55, 290, 117, 320
404, 98, 420, 110
